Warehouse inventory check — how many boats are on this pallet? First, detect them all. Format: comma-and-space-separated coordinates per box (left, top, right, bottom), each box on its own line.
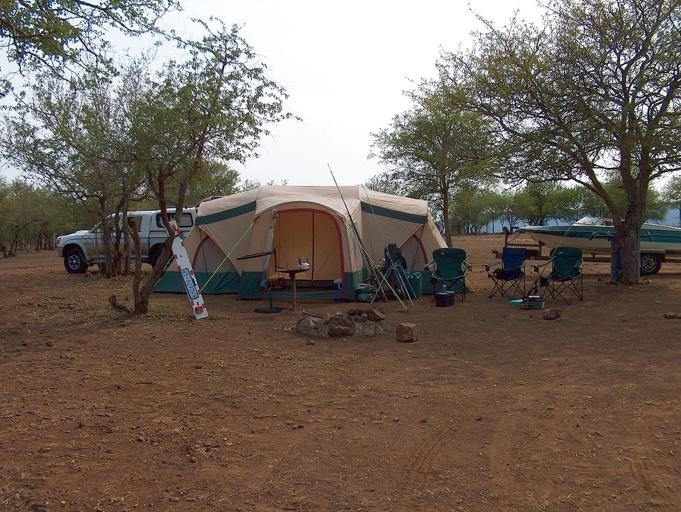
513, 214, 681, 256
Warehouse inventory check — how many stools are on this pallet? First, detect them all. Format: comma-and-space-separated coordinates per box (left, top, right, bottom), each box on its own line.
255, 286, 285, 315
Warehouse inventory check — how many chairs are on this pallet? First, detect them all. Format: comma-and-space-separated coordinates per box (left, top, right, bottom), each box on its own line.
482, 247, 529, 302
533, 246, 585, 305
430, 247, 467, 301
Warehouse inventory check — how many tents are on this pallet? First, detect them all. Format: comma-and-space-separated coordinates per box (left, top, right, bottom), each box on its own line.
150, 176, 477, 302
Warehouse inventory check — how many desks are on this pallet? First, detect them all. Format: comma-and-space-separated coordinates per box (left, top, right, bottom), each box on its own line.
276, 267, 309, 311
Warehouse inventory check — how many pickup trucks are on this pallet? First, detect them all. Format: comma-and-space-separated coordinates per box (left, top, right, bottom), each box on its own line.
52, 205, 199, 275
